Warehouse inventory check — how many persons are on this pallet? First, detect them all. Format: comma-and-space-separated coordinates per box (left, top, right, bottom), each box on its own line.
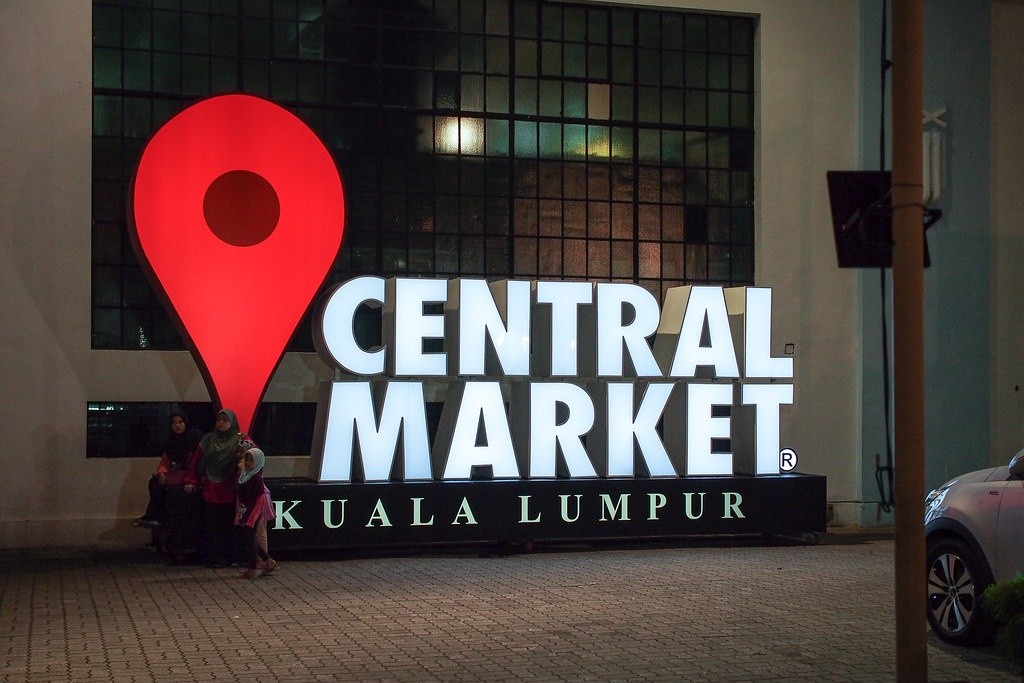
234, 448, 276, 575
132, 409, 257, 567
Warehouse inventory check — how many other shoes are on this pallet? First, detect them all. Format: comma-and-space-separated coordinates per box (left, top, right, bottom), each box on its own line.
130, 518, 163, 528
239, 570, 257, 579
212, 560, 232, 568
263, 562, 280, 573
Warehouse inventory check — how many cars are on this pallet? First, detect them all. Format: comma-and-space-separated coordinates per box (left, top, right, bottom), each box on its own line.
924, 451, 1024, 642
